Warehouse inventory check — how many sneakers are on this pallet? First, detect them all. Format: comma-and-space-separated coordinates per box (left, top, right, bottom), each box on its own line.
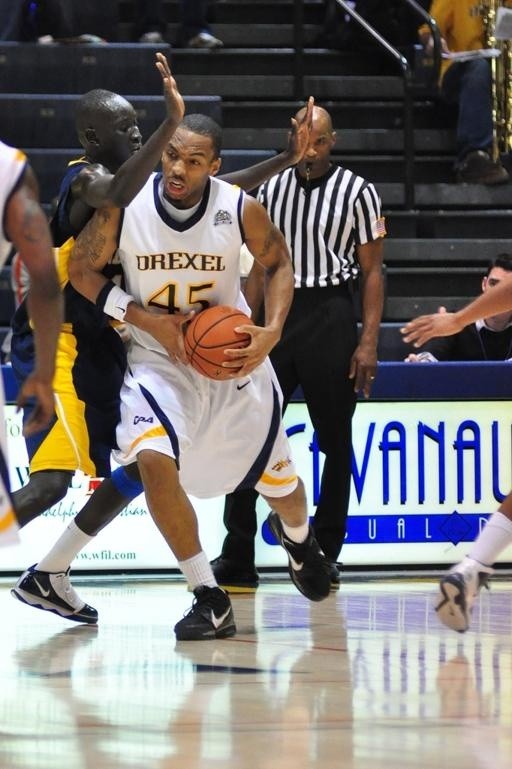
12, 564, 96, 623
434, 556, 494, 633
175, 509, 340, 640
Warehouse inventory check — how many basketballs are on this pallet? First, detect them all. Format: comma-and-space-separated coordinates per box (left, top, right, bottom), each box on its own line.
184, 306, 254, 381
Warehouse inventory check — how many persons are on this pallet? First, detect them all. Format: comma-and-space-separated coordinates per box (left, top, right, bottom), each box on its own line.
9, 52, 314, 624
135, 0, 223, 48
28, 0, 116, 44
402, 252, 512, 363
418, 0, 512, 186
67, 114, 331, 643
0, 140, 64, 437
401, 273, 512, 635
209, 105, 388, 590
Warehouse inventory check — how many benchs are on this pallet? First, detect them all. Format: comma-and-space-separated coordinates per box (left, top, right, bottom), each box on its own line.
0, 0, 511, 360
